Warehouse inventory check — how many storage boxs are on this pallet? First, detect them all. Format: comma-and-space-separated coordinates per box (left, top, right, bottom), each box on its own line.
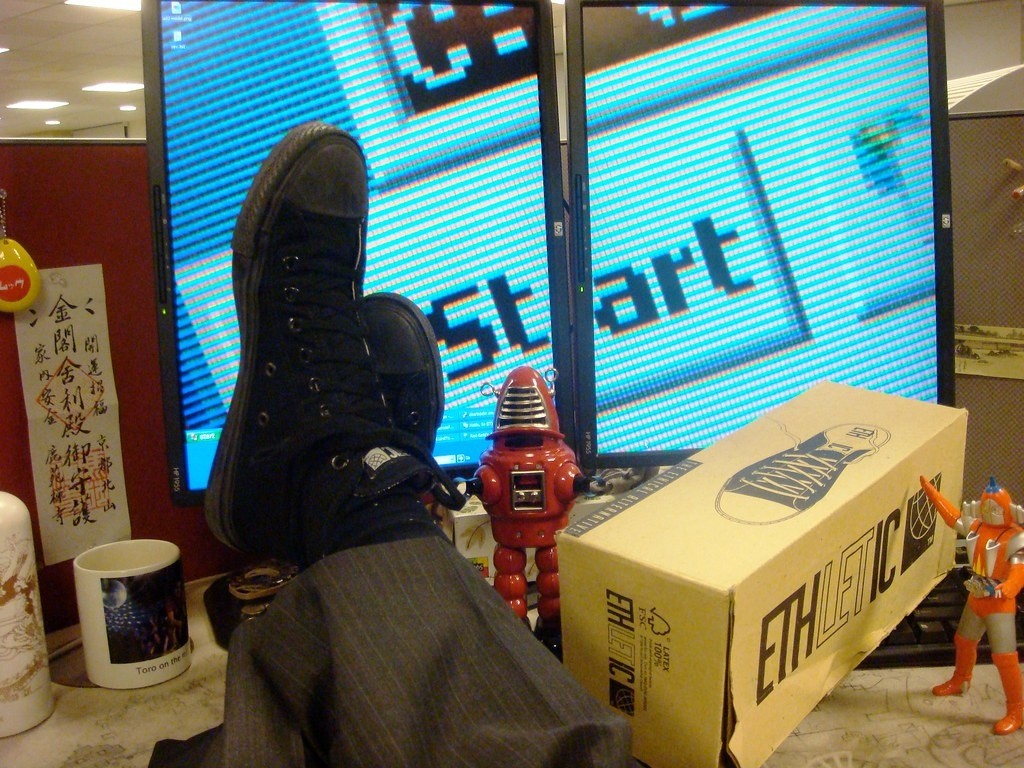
441, 487, 631, 586
554, 380, 971, 767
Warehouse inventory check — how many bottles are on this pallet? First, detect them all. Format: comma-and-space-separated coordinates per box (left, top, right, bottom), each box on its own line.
0, 490, 58, 738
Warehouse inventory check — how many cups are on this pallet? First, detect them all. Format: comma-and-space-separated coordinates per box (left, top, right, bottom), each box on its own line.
73, 538, 192, 690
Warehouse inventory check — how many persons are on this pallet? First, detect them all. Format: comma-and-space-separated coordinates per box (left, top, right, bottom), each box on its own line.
919, 474, 1024, 734
148, 123, 632, 767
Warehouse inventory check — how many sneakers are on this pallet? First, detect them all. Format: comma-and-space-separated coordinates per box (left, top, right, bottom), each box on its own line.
361, 292, 446, 453
204, 119, 467, 564
